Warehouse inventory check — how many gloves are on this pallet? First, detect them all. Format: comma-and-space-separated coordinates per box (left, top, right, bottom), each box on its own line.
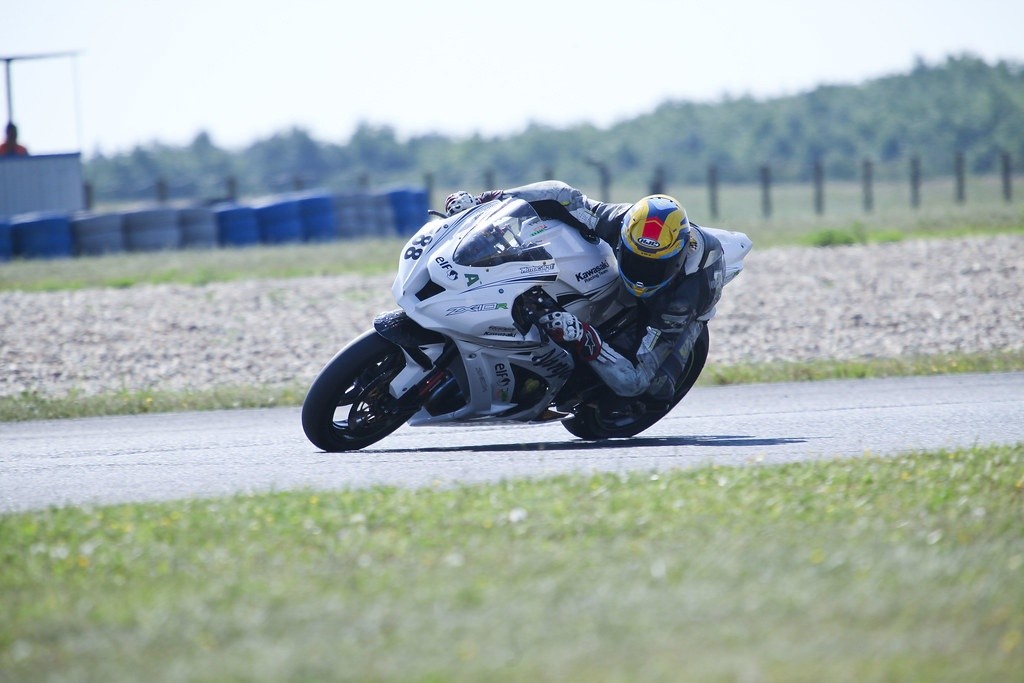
538, 310, 603, 359
445, 190, 504, 217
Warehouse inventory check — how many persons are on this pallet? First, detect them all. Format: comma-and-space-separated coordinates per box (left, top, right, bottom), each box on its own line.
445, 182, 726, 412
0, 122, 29, 156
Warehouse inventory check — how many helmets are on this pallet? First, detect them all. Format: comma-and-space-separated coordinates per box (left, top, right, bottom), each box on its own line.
616, 194, 691, 299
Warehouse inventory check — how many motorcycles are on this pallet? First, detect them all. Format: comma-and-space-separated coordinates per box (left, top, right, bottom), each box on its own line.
301, 192, 753, 453
